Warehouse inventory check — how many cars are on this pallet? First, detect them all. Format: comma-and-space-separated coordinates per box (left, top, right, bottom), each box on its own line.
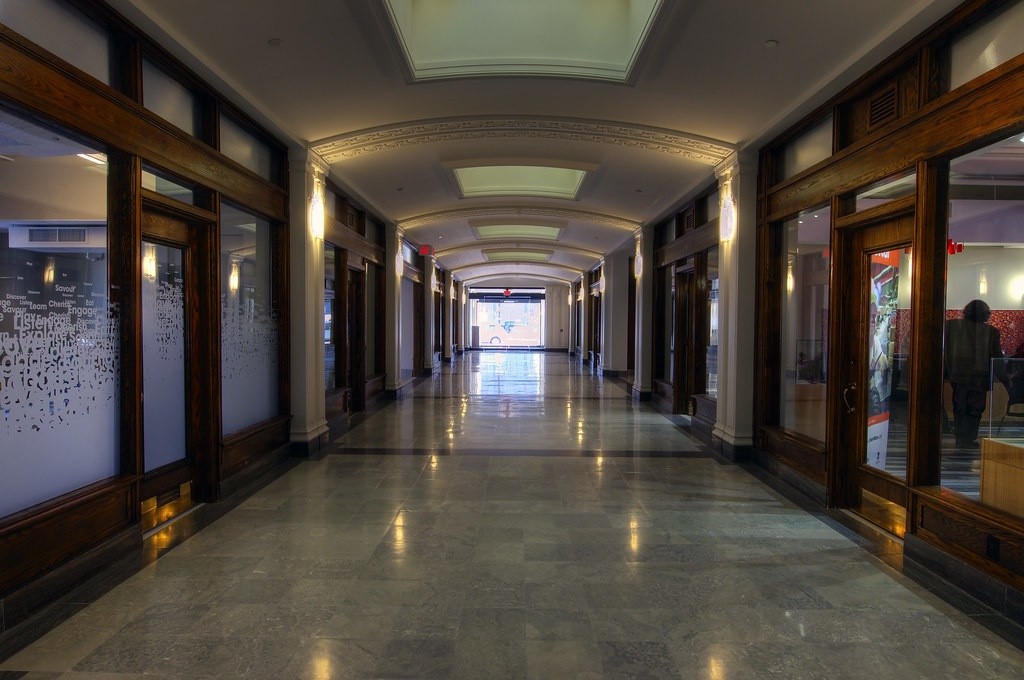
478, 323, 508, 346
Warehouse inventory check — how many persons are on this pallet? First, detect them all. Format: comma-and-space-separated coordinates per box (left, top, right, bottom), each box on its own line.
1006, 340, 1024, 403
943, 300, 1013, 448
